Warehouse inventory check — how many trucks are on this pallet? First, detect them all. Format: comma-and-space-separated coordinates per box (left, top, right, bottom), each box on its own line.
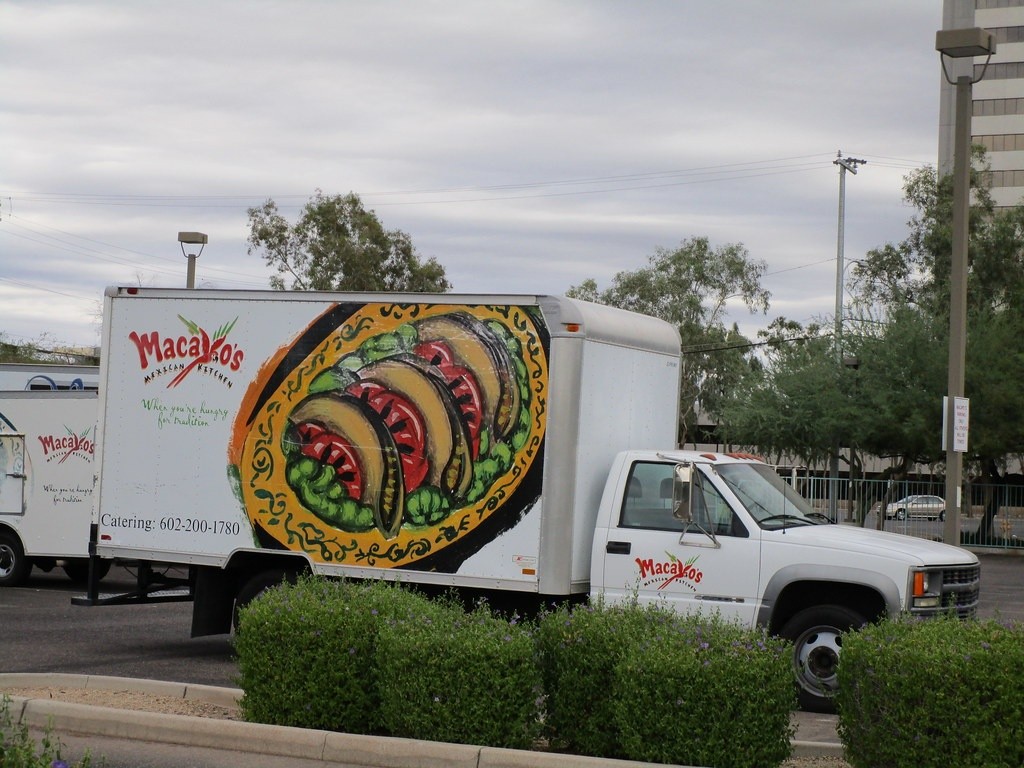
1, 389, 113, 584
91, 285, 984, 714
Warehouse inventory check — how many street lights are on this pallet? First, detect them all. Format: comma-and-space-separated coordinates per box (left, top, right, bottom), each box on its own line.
177, 230, 209, 290
935, 26, 998, 557
845, 355, 862, 521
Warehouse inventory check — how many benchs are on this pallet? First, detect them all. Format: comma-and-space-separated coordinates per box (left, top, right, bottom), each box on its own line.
625, 476, 673, 528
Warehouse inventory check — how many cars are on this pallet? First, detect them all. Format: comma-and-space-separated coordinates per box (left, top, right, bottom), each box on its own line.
876, 495, 946, 522
